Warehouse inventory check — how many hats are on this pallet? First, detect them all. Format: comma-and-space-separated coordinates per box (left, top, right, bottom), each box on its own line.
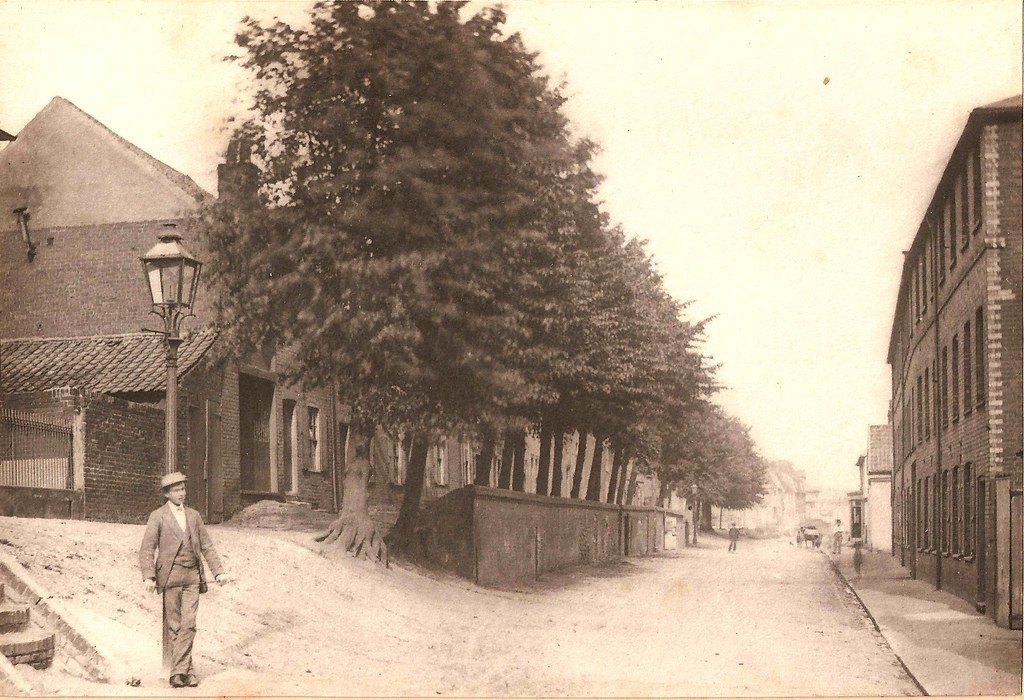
159, 472, 189, 490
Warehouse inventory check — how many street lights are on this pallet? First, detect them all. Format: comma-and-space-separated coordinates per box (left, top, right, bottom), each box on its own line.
138, 221, 206, 489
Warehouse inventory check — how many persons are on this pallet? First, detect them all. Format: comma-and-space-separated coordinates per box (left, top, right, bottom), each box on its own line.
796, 525, 823, 549
832, 520, 843, 554
728, 523, 740, 551
138, 471, 230, 687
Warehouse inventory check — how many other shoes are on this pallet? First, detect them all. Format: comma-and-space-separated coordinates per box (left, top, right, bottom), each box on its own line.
169, 675, 184, 687
184, 674, 198, 687
